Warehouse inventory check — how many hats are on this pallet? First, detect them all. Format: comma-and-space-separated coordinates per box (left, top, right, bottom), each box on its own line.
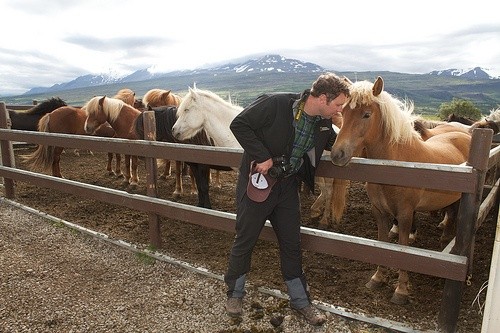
247, 160, 279, 202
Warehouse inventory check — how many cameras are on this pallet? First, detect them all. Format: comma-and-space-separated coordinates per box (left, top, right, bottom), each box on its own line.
267, 156, 291, 178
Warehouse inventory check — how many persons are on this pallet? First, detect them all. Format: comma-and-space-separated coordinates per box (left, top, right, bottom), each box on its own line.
223, 72, 350, 327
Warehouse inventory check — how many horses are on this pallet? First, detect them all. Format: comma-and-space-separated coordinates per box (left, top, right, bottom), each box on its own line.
83, 95, 156, 191
328, 76, 491, 306
131, 102, 233, 212
132, 89, 180, 109
18, 89, 134, 181
171, 84, 352, 231
5, 95, 65, 134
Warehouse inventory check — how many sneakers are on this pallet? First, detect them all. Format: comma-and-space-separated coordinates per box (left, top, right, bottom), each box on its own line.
291, 302, 326, 325
226, 296, 242, 317
